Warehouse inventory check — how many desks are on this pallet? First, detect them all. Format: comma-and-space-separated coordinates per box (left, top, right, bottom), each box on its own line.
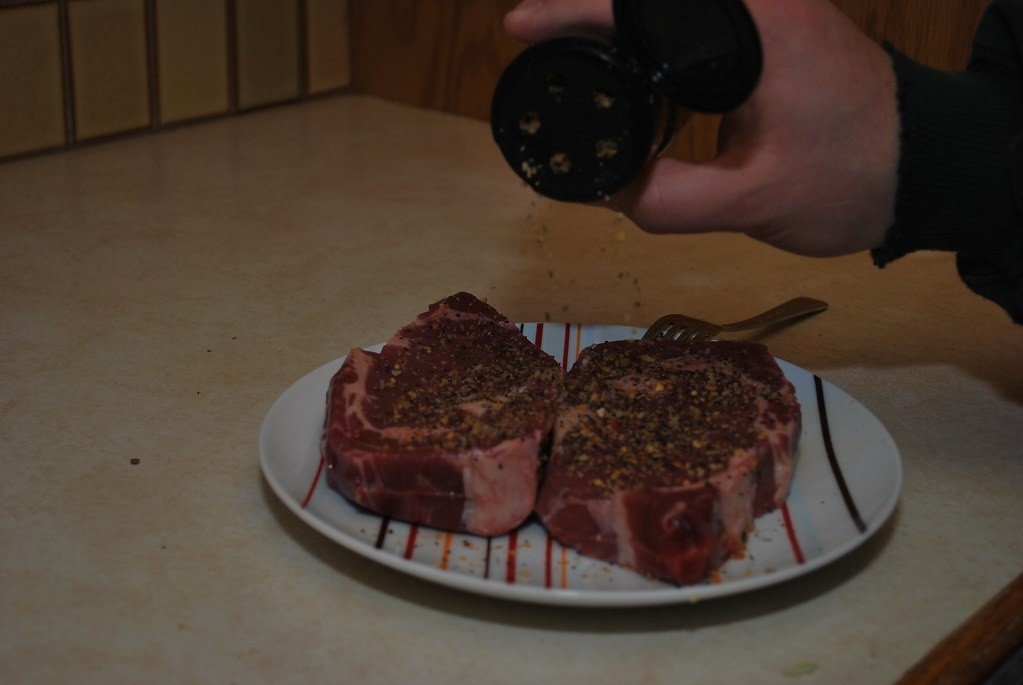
0, 92, 1023, 685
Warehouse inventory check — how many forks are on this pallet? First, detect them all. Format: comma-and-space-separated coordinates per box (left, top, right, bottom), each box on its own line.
641, 296, 829, 342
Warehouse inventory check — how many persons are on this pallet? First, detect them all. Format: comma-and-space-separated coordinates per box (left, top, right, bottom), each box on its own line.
504, 0, 1023, 320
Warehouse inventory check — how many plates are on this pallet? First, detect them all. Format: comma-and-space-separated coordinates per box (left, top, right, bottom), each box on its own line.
257, 320, 904, 610
